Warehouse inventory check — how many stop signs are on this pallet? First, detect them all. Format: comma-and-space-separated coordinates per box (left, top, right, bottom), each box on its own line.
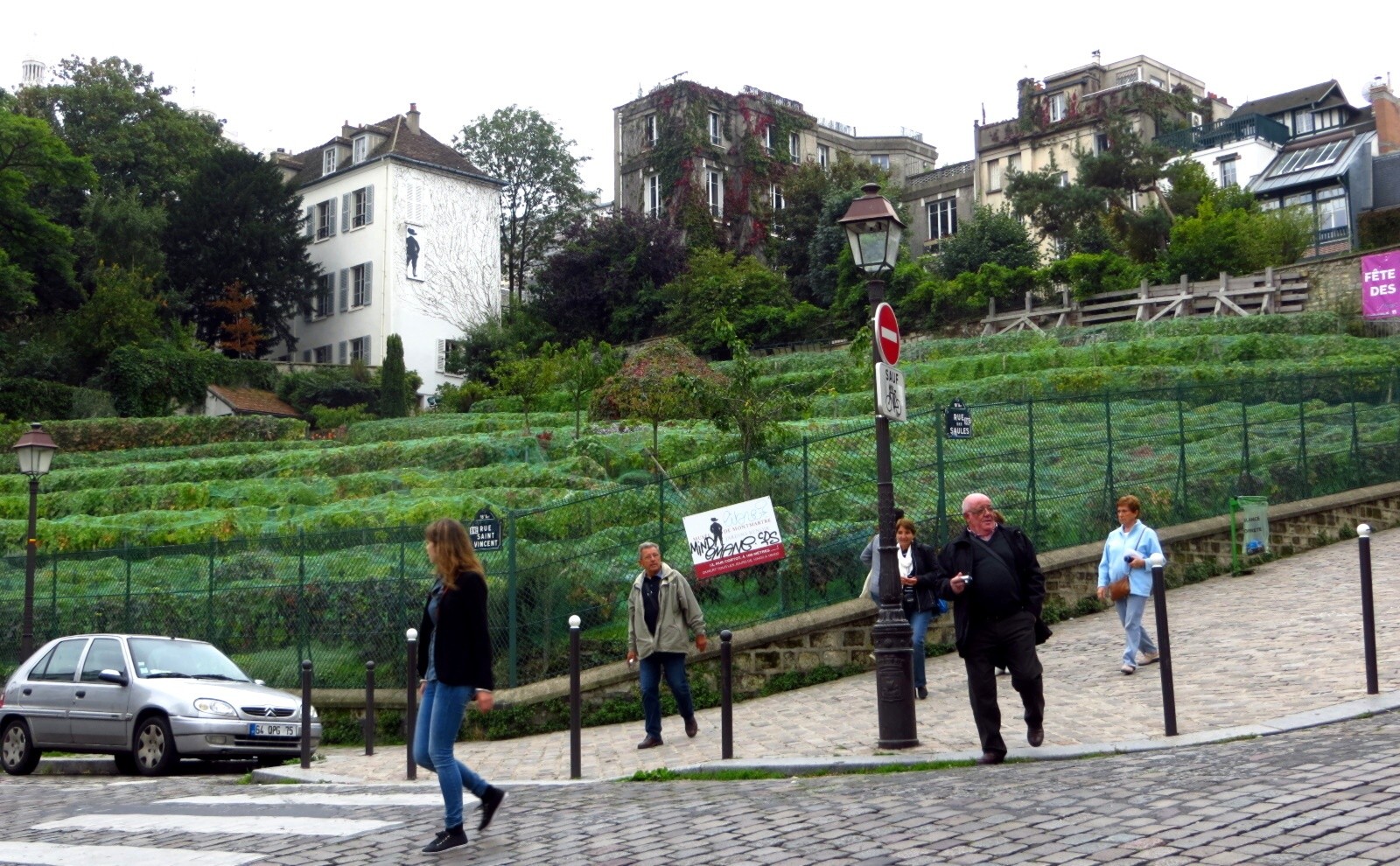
873, 302, 901, 366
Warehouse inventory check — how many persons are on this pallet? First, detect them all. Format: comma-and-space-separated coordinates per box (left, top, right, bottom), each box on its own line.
1097, 495, 1167, 673
993, 512, 1010, 675
860, 508, 905, 660
896, 519, 939, 700
935, 493, 1051, 764
413, 518, 509, 855
628, 542, 707, 749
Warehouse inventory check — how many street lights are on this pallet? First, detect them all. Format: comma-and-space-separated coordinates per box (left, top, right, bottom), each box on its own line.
836, 184, 919, 748
11, 421, 59, 667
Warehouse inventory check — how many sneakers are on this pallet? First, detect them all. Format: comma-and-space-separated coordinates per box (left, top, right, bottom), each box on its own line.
638, 735, 663, 749
476, 788, 509, 832
685, 714, 698, 738
420, 831, 469, 854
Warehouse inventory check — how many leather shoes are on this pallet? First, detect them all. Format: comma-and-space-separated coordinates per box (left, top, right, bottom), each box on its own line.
1027, 722, 1044, 747
978, 752, 1002, 761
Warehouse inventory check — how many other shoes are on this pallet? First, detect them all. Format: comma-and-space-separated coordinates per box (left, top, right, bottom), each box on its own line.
1138, 650, 1159, 665
868, 651, 876, 662
917, 684, 928, 699
1121, 663, 1134, 673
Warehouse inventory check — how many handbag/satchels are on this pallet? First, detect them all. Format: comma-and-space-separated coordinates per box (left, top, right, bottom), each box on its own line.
1035, 616, 1053, 645
859, 568, 874, 600
1109, 575, 1130, 600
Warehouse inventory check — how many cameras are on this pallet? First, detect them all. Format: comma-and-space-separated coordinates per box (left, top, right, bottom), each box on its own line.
1124, 556, 1132, 562
960, 575, 973, 583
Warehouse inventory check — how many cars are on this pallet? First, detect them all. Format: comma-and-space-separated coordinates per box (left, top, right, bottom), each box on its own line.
1, 633, 322, 778
